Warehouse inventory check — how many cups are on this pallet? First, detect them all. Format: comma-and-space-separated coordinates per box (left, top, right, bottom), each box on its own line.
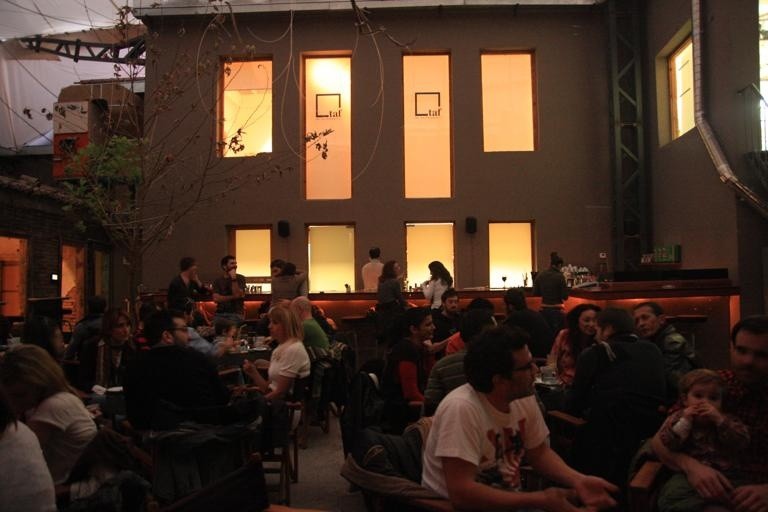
247, 336, 255, 347
539, 366, 553, 381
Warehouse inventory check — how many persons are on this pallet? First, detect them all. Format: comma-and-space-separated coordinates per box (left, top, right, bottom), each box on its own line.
360, 247, 767, 509
1, 250, 336, 511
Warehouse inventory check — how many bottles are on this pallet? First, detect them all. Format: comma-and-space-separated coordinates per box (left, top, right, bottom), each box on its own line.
560, 264, 595, 288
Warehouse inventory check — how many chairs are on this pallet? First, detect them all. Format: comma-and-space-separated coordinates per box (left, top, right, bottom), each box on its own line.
0, 295, 768, 512
